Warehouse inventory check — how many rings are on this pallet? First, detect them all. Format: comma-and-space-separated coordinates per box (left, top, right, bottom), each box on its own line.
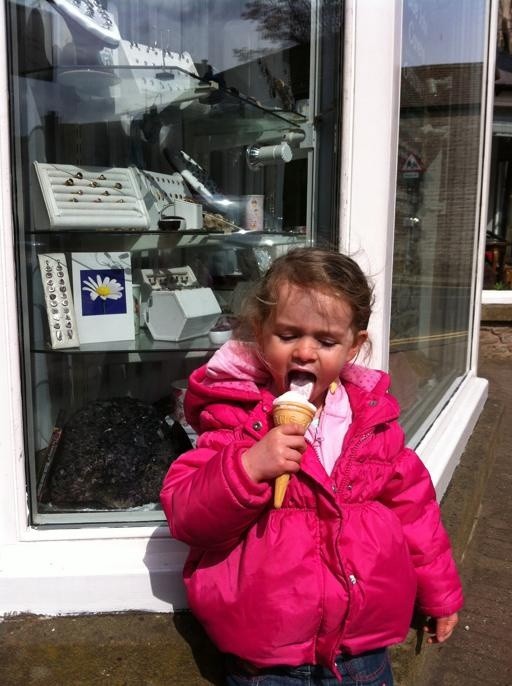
65, 171, 124, 203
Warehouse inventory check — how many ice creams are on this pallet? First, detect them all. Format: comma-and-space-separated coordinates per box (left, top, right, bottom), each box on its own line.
273, 383, 319, 512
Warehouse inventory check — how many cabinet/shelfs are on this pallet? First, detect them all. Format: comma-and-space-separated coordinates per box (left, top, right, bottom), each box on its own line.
5, 0, 320, 526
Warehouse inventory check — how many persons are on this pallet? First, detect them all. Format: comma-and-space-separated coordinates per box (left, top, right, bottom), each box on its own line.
158, 247, 465, 686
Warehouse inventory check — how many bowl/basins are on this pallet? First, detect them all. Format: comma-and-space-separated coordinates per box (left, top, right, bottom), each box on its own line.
207, 329, 233, 345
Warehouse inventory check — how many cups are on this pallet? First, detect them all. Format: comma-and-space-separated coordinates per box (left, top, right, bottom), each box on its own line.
245, 194, 265, 230
